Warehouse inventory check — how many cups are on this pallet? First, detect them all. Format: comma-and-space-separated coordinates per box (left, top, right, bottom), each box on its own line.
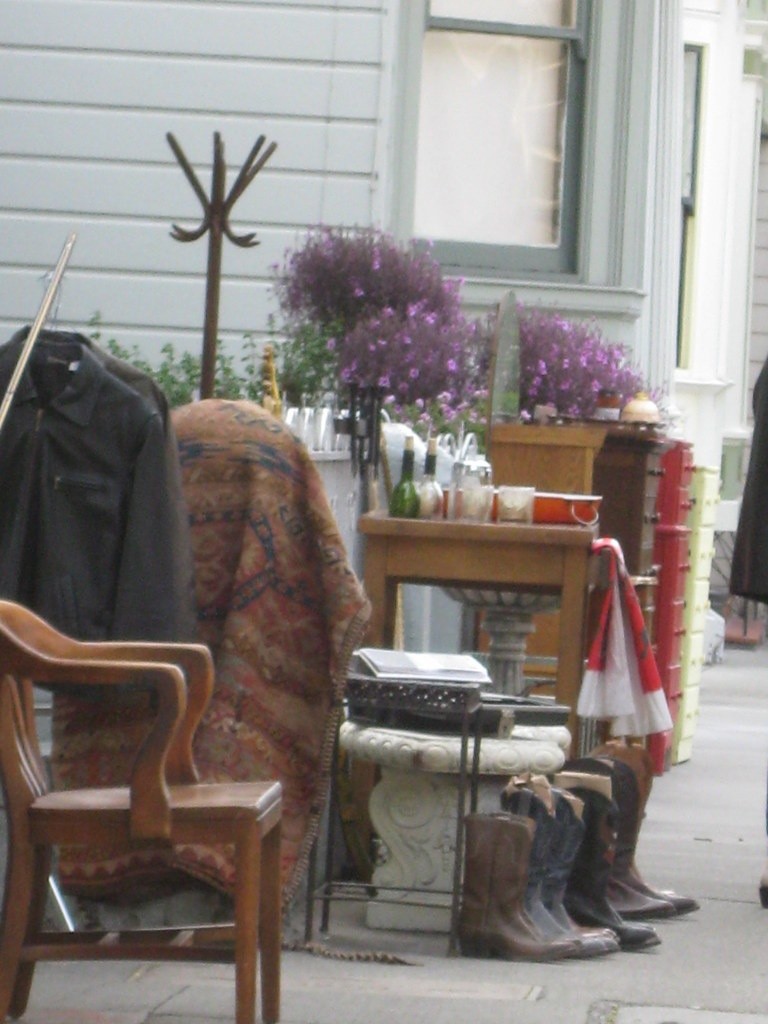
497, 486, 536, 524
447, 462, 493, 525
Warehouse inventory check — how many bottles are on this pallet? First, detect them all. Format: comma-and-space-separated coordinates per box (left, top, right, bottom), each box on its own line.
390, 434, 419, 518
416, 438, 444, 521
594, 389, 621, 422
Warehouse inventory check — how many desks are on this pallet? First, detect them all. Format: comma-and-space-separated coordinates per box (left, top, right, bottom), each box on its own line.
360, 508, 602, 834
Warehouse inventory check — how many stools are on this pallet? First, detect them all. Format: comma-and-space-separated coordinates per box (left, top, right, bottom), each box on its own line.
338, 722, 572, 933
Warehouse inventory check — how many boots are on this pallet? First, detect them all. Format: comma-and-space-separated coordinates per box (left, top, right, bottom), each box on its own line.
460, 755, 701, 964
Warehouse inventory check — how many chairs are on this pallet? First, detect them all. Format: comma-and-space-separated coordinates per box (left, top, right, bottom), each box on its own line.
0, 599, 286, 1024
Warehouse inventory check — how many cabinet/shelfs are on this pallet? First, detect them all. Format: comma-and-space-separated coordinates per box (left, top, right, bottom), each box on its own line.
475, 420, 670, 760
647, 436, 723, 776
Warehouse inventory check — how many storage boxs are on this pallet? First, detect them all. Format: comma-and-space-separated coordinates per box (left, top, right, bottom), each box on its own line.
346, 678, 482, 734
442, 490, 604, 527
